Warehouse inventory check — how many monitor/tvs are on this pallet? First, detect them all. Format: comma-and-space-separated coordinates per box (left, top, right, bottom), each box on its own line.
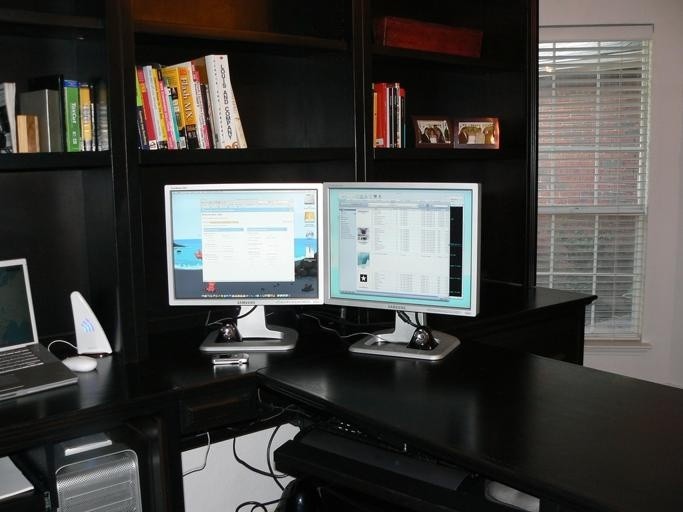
324, 182, 482, 360
164, 183, 324, 352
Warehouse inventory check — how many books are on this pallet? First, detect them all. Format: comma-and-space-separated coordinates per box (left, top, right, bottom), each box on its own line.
134, 54, 249, 150
0, 76, 111, 154
371, 81, 411, 150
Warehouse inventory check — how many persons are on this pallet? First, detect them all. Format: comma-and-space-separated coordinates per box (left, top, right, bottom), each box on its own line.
417, 124, 496, 146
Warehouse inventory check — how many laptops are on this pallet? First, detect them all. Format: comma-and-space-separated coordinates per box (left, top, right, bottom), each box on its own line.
0, 258, 79, 402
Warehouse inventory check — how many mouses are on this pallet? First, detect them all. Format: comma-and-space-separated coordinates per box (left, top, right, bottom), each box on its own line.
62, 356, 98, 372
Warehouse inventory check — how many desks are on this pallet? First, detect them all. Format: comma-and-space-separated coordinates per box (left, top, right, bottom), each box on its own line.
0, 288, 683, 512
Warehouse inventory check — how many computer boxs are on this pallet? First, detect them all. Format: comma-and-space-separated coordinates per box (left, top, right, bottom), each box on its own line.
27, 432, 142, 512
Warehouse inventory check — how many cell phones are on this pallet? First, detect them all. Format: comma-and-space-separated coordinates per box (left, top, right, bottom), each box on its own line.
211, 353, 250, 364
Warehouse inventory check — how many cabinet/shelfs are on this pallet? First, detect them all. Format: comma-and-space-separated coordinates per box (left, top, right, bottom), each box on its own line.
123, 1, 364, 363
360, 0, 537, 289
1, 0, 136, 364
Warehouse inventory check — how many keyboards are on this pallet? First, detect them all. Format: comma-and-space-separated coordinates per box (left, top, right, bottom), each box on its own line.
318, 417, 461, 468
